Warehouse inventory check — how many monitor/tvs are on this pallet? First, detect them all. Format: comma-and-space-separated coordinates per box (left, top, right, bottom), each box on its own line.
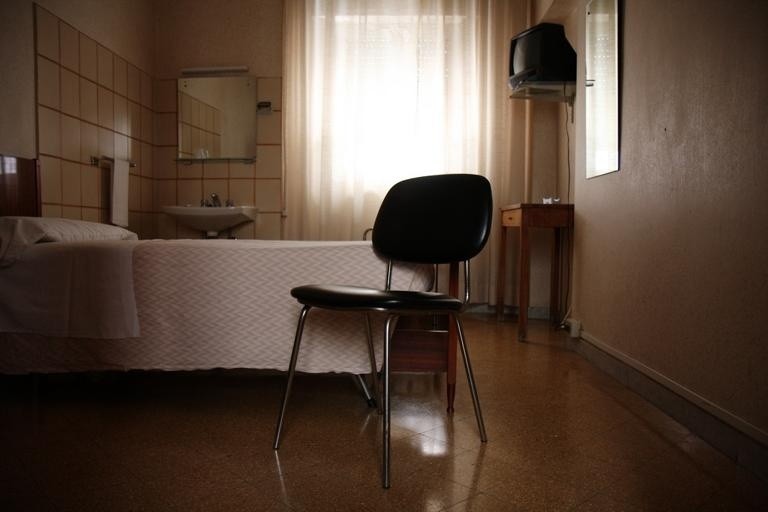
509, 23, 578, 90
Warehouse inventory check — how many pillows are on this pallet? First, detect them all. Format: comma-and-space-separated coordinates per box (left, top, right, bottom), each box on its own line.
0, 216, 138, 242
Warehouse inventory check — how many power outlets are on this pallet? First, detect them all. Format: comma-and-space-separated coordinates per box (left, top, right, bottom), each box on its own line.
567, 319, 579, 338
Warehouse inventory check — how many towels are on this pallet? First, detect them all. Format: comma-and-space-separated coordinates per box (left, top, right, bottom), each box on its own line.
110, 158, 129, 227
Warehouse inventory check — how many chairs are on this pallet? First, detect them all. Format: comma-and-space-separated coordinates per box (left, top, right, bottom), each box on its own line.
273, 174, 493, 490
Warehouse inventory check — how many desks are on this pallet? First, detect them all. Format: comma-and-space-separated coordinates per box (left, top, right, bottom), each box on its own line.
496, 202, 574, 343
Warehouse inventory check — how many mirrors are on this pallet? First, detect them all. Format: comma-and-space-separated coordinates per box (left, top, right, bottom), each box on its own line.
178, 74, 256, 162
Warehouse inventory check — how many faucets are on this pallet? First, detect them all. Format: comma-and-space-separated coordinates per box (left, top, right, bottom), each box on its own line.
209, 192, 222, 207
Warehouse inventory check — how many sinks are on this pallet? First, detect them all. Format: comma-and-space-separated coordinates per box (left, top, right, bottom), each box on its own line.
159, 206, 259, 237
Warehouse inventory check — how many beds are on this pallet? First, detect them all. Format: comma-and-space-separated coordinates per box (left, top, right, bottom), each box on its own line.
0, 155, 458, 414
